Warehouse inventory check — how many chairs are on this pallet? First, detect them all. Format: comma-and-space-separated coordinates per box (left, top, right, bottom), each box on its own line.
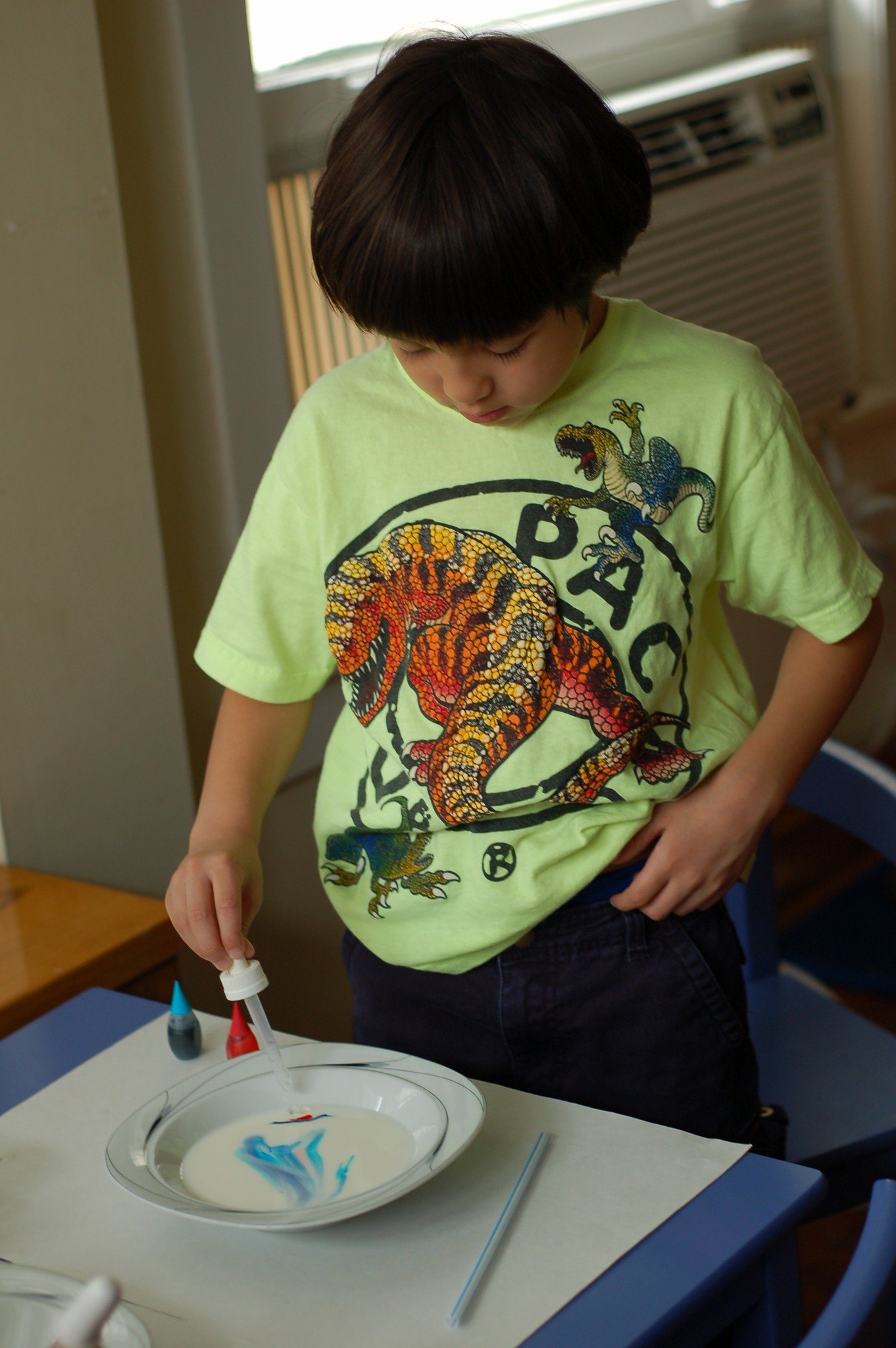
719, 746, 896, 1348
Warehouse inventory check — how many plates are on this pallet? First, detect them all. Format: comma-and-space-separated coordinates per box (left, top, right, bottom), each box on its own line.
0, 1265, 152, 1348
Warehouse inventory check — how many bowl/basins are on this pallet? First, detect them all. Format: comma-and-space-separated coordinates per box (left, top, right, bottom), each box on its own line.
104, 1039, 487, 1236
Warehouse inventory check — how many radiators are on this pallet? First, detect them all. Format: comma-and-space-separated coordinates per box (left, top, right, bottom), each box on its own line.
554, 48, 870, 449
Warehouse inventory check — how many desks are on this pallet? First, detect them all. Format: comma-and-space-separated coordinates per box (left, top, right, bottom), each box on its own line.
1, 984, 826, 1346
1, 865, 188, 1046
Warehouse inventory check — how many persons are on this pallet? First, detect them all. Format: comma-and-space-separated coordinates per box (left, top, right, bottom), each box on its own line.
164, 32, 885, 1159
